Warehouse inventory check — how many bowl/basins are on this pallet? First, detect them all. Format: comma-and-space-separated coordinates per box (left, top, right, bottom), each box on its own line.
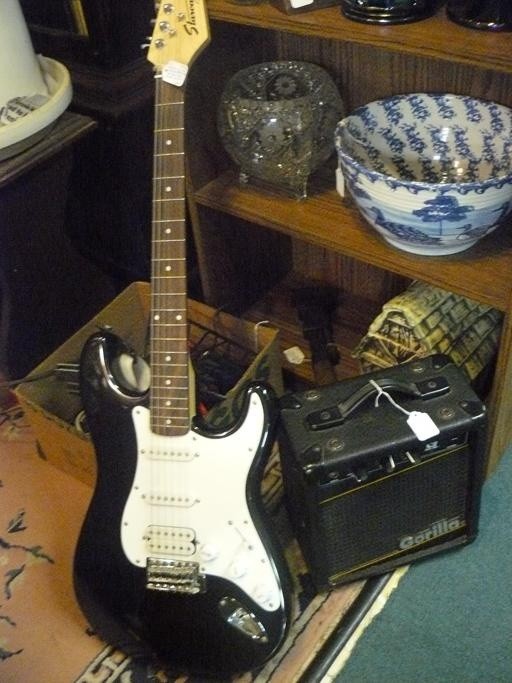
329, 90, 512, 259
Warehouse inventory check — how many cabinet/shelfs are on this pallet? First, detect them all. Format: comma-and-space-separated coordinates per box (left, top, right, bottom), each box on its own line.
39, 30, 175, 283
1, 108, 101, 385
184, 0, 512, 478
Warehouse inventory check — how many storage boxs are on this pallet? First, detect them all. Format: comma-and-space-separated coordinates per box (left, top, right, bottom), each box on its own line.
9, 277, 289, 497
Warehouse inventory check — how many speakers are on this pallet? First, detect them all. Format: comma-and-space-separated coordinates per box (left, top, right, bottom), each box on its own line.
275, 354, 489, 592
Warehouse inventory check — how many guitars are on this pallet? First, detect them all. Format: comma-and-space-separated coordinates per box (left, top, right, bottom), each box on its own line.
74, 1, 298, 677
